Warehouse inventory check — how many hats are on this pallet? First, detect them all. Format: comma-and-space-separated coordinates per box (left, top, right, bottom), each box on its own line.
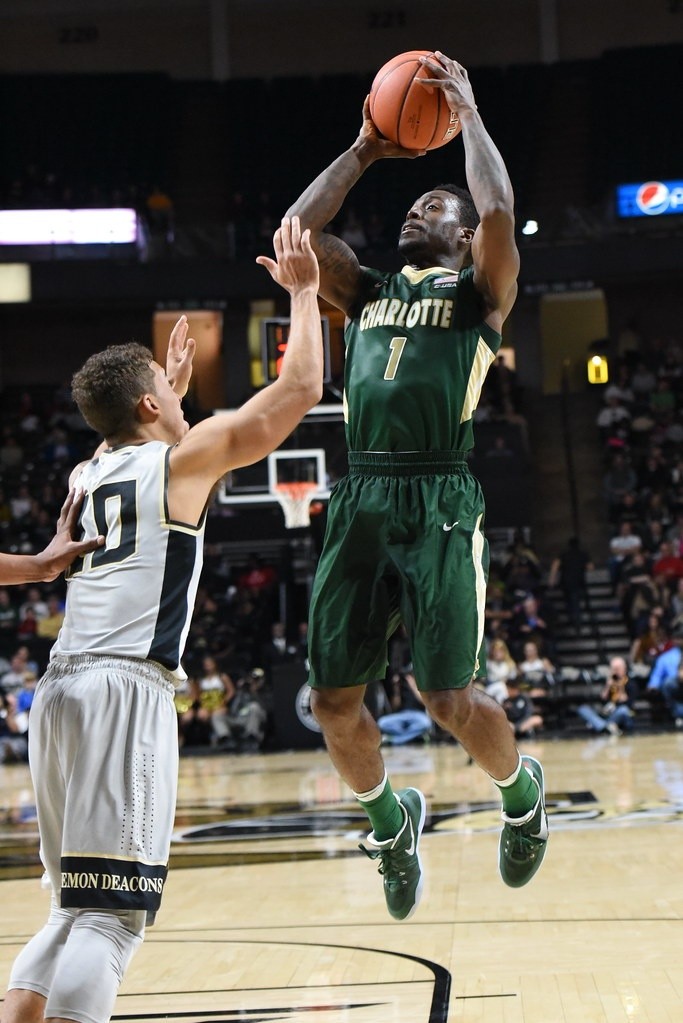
249, 668, 265, 679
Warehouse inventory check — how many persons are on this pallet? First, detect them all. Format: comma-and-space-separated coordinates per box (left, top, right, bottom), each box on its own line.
281, 51, 549, 921
1, 216, 324, 1023
0, 351, 683, 763
0, 486, 106, 586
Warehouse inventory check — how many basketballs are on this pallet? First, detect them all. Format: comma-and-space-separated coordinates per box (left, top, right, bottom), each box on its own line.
367, 48, 463, 150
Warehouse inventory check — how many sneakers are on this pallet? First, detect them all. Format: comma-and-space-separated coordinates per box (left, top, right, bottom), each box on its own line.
359, 787, 426, 920
499, 754, 548, 887
